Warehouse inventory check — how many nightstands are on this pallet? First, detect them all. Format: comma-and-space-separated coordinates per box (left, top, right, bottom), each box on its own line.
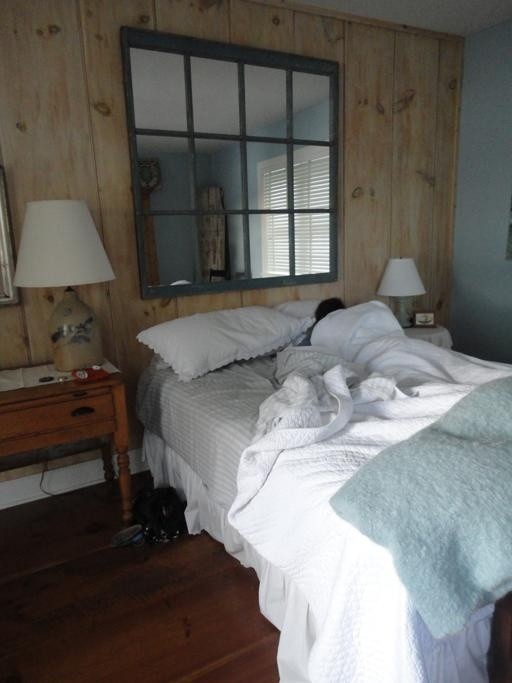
1, 358, 136, 518
405, 323, 454, 349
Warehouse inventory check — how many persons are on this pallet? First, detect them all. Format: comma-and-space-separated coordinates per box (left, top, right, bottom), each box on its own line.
295, 298, 346, 346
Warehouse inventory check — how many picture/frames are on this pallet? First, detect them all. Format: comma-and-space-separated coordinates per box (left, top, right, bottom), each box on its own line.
413, 311, 436, 329
0, 157, 19, 306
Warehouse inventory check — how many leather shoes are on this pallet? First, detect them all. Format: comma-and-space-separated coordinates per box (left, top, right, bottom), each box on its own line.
133, 485, 185, 545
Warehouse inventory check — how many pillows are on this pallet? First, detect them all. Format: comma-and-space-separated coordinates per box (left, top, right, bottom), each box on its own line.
136, 307, 316, 383
276, 301, 318, 319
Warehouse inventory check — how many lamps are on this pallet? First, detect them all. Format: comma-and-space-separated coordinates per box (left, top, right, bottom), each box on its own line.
14, 200, 118, 370
377, 257, 427, 328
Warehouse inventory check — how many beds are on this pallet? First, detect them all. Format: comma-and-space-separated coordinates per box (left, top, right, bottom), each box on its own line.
128, 299, 512, 683
